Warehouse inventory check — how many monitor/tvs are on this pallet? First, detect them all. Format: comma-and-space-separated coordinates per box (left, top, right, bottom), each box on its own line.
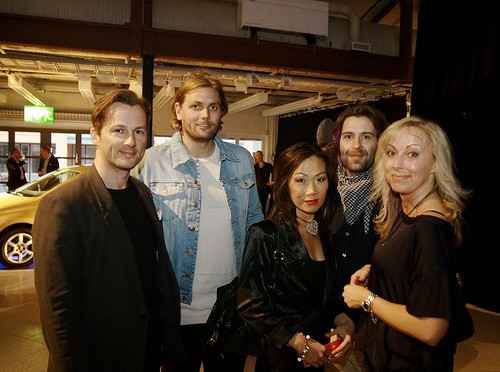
23, 105, 54, 123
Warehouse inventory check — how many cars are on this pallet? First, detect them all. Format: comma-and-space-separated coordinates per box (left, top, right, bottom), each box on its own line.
0, 165, 94, 269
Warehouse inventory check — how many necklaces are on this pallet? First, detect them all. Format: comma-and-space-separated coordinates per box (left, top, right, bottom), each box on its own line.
297, 215, 319, 236
382, 187, 436, 246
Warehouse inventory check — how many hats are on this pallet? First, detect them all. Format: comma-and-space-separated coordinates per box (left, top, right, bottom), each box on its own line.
316, 119, 335, 145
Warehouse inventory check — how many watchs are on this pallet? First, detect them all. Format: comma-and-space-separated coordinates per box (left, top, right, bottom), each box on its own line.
361, 293, 377, 311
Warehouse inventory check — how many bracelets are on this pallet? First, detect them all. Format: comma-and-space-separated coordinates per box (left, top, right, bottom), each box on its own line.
297, 335, 310, 362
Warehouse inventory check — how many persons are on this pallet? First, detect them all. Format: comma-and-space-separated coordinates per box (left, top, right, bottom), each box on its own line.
130, 71, 264, 372
6, 148, 28, 194
38, 145, 59, 176
31, 89, 182, 372
238, 144, 361, 372
254, 151, 275, 216
330, 105, 388, 276
342, 116, 474, 372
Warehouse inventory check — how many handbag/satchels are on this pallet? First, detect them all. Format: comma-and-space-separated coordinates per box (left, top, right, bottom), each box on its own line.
207, 219, 278, 357
451, 270, 474, 345
